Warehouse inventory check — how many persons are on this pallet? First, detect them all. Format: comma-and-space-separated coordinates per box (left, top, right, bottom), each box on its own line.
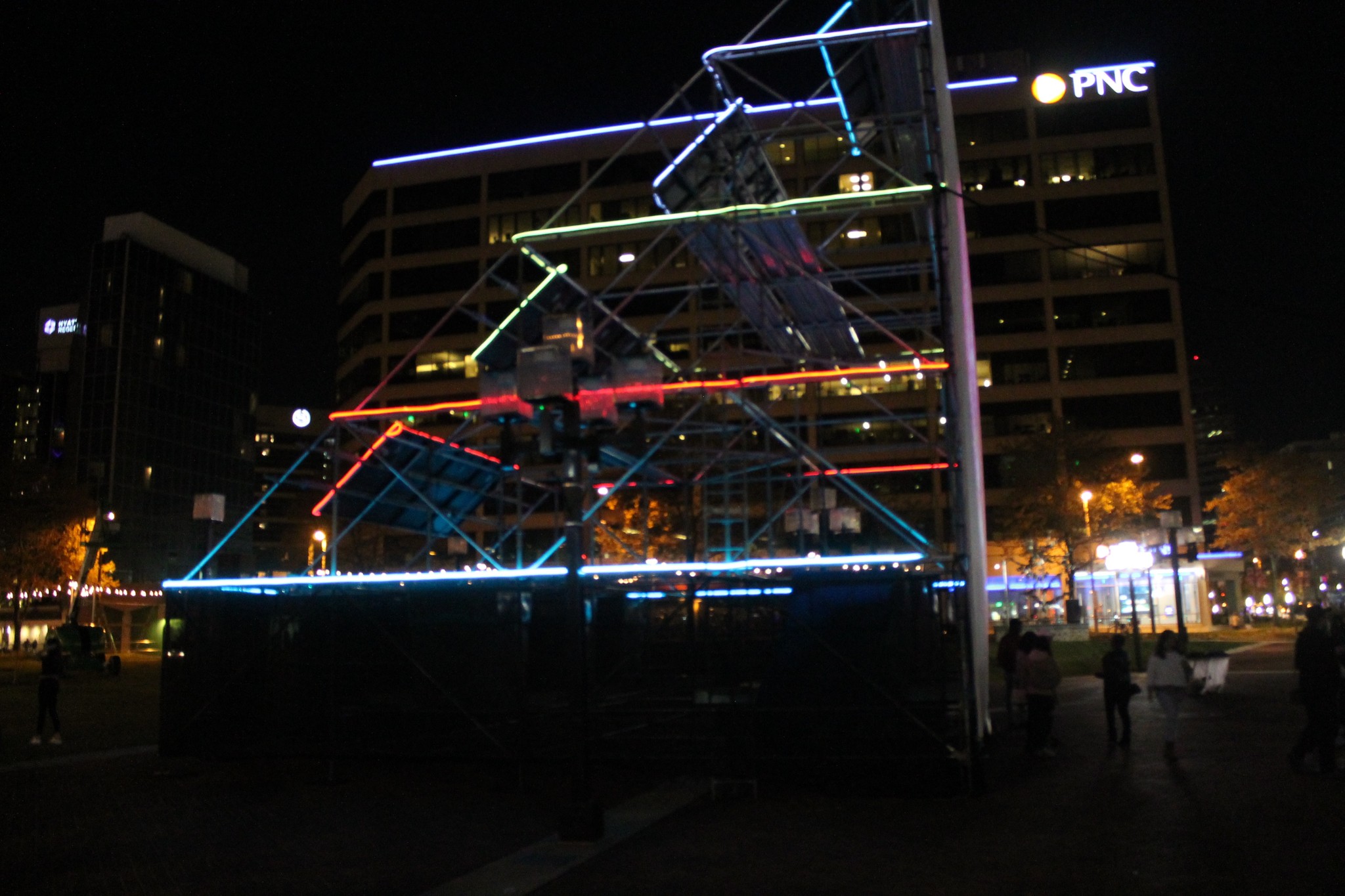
1094, 634, 1133, 754
1145, 629, 1193, 762
30, 638, 63, 746
1287, 605, 1345, 776
998, 618, 1059, 758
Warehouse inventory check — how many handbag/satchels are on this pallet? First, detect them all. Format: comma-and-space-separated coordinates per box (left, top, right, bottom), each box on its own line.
1011, 689, 1029, 724
1127, 683, 1140, 695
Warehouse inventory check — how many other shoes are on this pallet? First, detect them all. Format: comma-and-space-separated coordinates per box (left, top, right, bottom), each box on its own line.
28, 738, 41, 745
51, 738, 63, 745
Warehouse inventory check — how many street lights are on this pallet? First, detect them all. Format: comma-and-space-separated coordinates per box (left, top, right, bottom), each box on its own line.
192, 493, 226, 580
516, 316, 665, 896
785, 489, 861, 558
1160, 510, 1188, 643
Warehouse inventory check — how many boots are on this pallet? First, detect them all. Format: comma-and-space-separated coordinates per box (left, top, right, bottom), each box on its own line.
1164, 741, 1179, 761
